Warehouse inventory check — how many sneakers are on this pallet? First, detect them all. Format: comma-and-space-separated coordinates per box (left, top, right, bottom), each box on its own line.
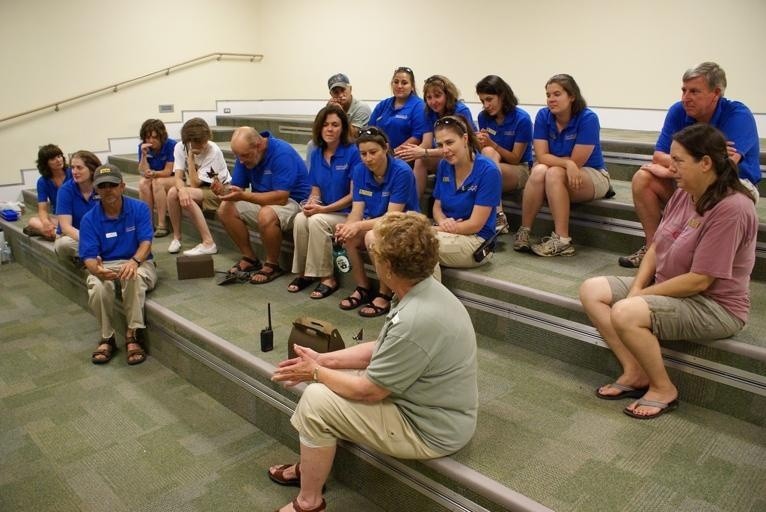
618, 244, 653, 269
513, 225, 533, 253
495, 211, 511, 235
182, 241, 219, 258
531, 231, 577, 258
167, 239, 182, 254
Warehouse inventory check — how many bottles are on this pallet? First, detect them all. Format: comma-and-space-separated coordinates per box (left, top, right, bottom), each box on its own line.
331, 238, 353, 274
0, 240, 13, 265
55, 223, 63, 241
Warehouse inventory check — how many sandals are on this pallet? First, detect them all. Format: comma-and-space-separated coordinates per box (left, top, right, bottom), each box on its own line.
122, 332, 150, 365
249, 261, 285, 286
359, 294, 394, 319
227, 256, 262, 278
88, 330, 119, 367
272, 495, 329, 512
266, 463, 329, 492
153, 222, 172, 238
339, 285, 372, 312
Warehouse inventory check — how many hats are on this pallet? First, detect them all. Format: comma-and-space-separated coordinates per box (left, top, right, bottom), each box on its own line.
327, 73, 350, 92
92, 164, 123, 187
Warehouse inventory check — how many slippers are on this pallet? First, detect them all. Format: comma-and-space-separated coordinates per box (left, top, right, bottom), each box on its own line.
596, 374, 652, 400
621, 396, 679, 420
309, 279, 341, 299
285, 275, 318, 294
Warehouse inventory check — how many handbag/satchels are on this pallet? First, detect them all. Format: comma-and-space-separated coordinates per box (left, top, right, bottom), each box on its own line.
287, 315, 347, 362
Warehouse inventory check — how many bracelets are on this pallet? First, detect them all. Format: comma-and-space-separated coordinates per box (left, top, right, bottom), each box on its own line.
132, 257, 140, 267
311, 364, 320, 381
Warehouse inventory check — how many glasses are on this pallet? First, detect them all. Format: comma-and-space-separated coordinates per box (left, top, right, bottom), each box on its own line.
432, 117, 466, 133
356, 129, 379, 135
396, 67, 412, 76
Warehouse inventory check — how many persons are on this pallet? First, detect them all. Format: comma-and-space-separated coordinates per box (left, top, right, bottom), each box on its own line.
617, 60, 761, 268
76, 162, 160, 366
21, 145, 72, 243
137, 104, 502, 316
302, 67, 615, 256
268, 208, 480, 512
577, 121, 759, 420
54, 151, 103, 267
